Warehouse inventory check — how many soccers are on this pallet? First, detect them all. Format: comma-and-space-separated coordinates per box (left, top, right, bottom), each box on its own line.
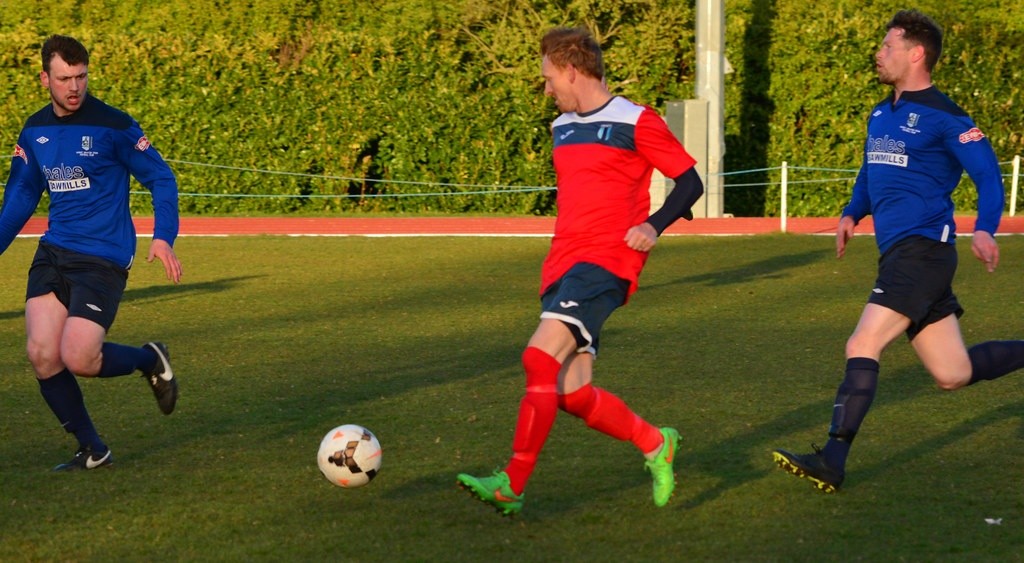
316, 424, 383, 490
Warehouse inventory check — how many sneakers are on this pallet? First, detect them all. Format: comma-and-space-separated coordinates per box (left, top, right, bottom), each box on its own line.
143, 342, 177, 415
771, 442, 845, 493
55, 443, 114, 474
643, 427, 684, 509
456, 470, 526, 519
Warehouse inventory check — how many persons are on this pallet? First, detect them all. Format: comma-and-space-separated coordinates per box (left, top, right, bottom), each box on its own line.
0, 34, 180, 470
456, 27, 704, 517
775, 10, 1024, 493
